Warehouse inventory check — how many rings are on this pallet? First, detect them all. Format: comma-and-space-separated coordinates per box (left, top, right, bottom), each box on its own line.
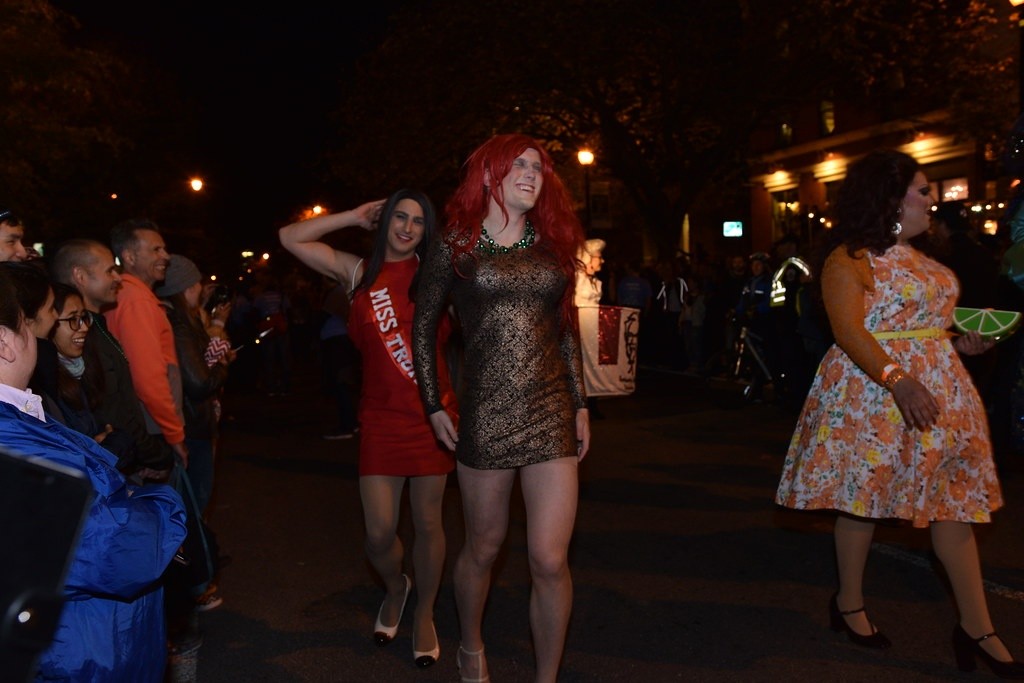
221, 307, 223, 309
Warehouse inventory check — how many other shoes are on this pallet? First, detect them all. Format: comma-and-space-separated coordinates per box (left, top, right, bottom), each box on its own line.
166, 632, 204, 657
172, 543, 188, 565
188, 580, 223, 613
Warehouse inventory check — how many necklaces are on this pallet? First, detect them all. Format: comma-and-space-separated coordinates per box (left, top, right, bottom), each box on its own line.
477, 219, 536, 254
94, 317, 123, 355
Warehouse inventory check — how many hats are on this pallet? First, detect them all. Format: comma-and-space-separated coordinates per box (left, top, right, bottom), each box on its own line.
152, 254, 203, 297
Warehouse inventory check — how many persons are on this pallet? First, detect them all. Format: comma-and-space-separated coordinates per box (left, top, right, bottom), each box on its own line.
0, 206, 236, 683
775, 153, 1024, 681
412, 134, 591, 683
199, 273, 361, 440
935, 201, 997, 275
598, 234, 813, 404
574, 239, 605, 306
278, 189, 460, 669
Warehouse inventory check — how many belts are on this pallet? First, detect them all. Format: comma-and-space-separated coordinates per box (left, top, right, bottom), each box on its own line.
872, 328, 946, 339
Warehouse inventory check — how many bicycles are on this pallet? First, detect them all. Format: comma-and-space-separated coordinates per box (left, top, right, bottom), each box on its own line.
702, 315, 816, 411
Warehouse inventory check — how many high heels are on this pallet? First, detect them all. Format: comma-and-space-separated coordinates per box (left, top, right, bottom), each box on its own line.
456, 641, 490, 683
412, 619, 440, 668
951, 621, 1024, 680
373, 573, 412, 648
829, 590, 893, 648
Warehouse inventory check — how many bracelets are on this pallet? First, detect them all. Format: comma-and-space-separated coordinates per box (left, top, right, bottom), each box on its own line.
213, 319, 224, 327
881, 364, 904, 389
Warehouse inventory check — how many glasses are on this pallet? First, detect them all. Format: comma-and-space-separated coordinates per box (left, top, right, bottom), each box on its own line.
57, 311, 93, 331
